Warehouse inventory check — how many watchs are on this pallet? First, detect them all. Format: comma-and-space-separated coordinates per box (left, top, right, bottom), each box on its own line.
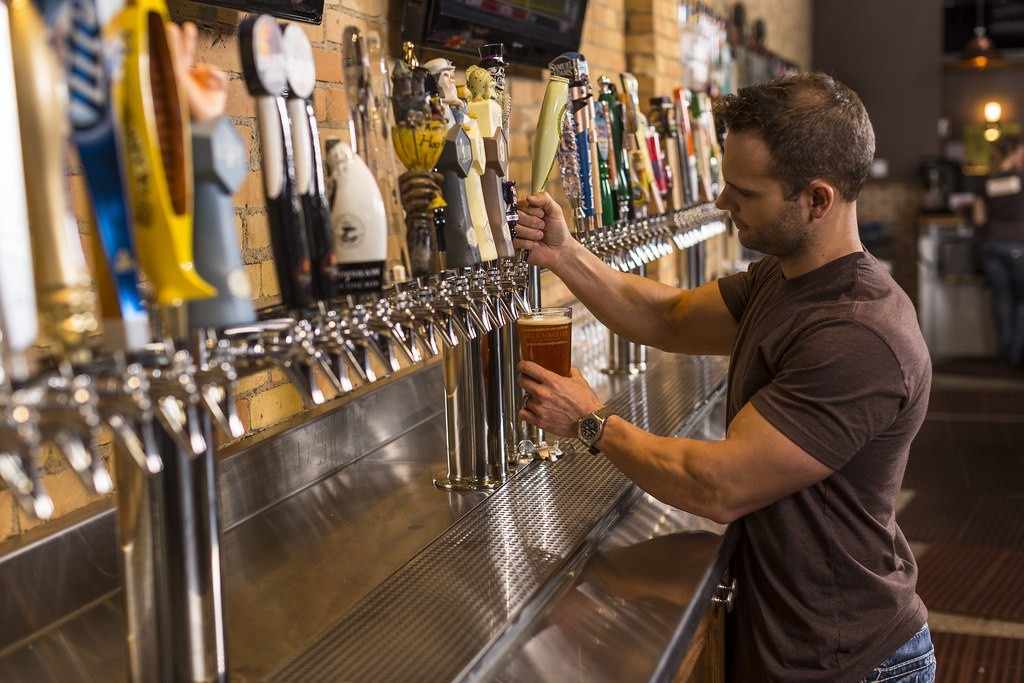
574, 405, 619, 456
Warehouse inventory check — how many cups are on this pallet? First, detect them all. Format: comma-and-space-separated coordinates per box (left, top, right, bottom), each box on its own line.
517, 307, 574, 377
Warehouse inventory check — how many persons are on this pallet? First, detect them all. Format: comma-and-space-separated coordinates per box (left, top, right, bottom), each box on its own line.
509, 70, 938, 682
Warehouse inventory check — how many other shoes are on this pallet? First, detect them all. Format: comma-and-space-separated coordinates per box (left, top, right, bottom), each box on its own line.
996, 357, 1024, 369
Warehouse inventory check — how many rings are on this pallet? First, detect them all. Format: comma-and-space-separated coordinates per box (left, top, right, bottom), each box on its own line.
521, 393, 531, 410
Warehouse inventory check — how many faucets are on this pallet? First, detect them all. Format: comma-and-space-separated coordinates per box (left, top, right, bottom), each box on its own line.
0, 197, 730, 523
